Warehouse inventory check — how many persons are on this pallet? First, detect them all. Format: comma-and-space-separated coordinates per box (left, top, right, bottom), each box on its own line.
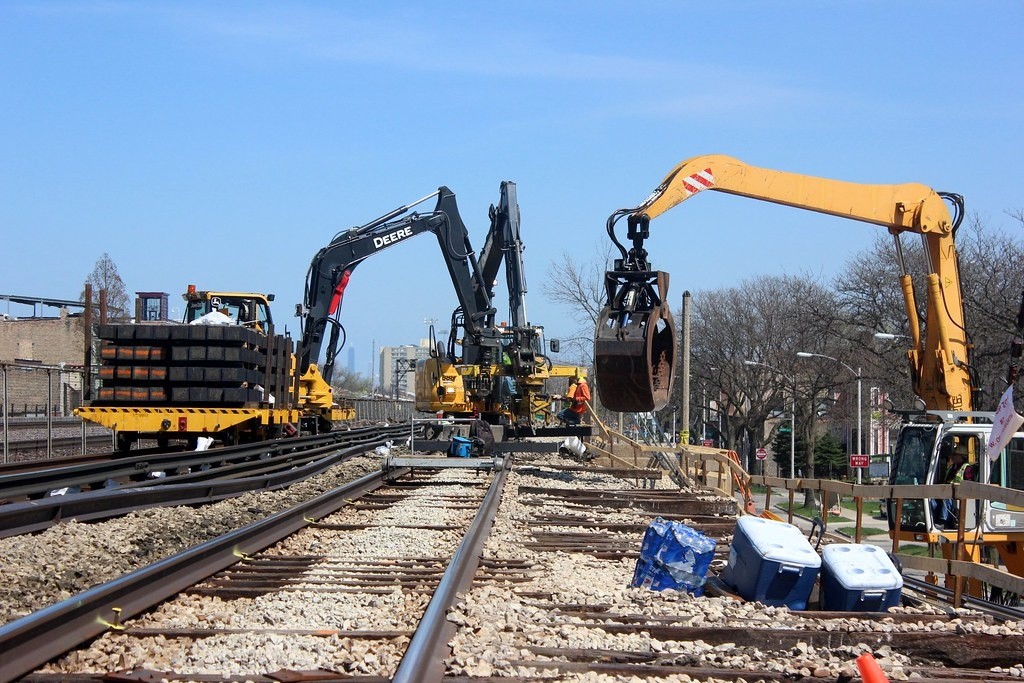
930, 446, 975, 530
503, 353, 516, 394
557, 376, 590, 425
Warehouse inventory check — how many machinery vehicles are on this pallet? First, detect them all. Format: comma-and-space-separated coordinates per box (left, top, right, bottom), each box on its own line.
75, 180, 593, 452
591, 153, 1024, 607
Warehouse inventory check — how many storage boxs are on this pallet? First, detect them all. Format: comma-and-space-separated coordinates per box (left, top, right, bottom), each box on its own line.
723, 515, 821, 610
449, 436, 472, 457
819, 541, 904, 612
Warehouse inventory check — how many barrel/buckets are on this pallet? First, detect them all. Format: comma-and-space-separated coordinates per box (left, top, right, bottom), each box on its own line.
564, 436, 586, 456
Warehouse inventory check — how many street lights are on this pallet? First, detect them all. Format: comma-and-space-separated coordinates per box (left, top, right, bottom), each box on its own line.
743, 361, 795, 479
796, 351, 862, 484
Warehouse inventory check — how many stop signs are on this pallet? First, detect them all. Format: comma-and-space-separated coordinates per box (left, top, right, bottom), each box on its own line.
756, 448, 768, 460
